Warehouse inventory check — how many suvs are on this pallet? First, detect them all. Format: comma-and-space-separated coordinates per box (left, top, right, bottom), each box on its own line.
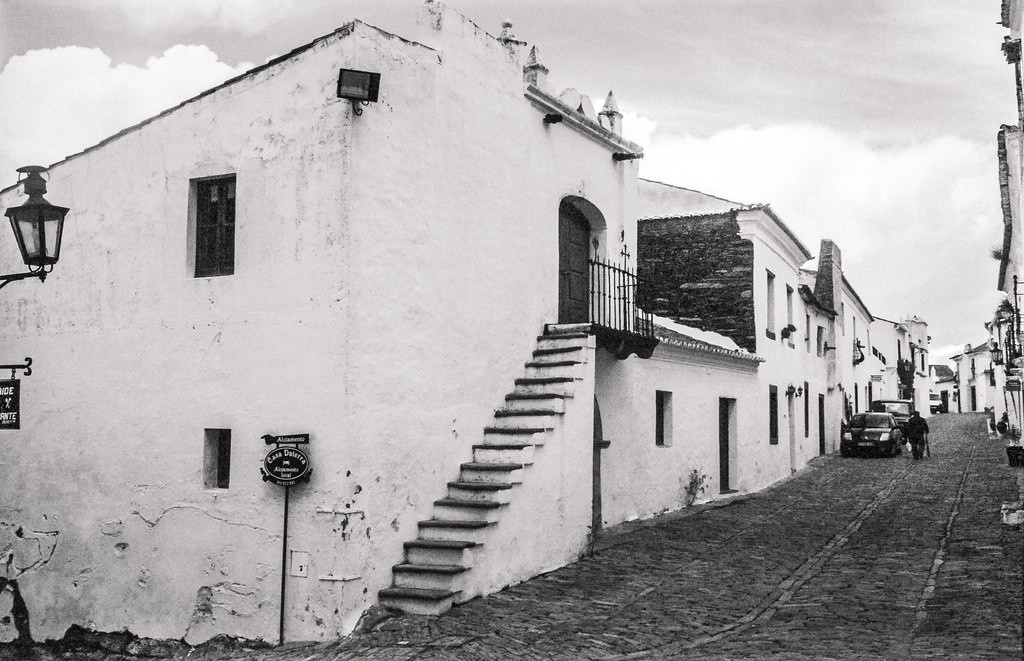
865, 398, 919, 440
928, 393, 945, 414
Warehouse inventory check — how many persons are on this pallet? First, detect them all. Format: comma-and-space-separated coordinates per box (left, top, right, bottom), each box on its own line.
906, 411, 929, 461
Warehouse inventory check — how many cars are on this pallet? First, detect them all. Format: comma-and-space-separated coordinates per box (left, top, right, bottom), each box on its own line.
840, 412, 903, 457
